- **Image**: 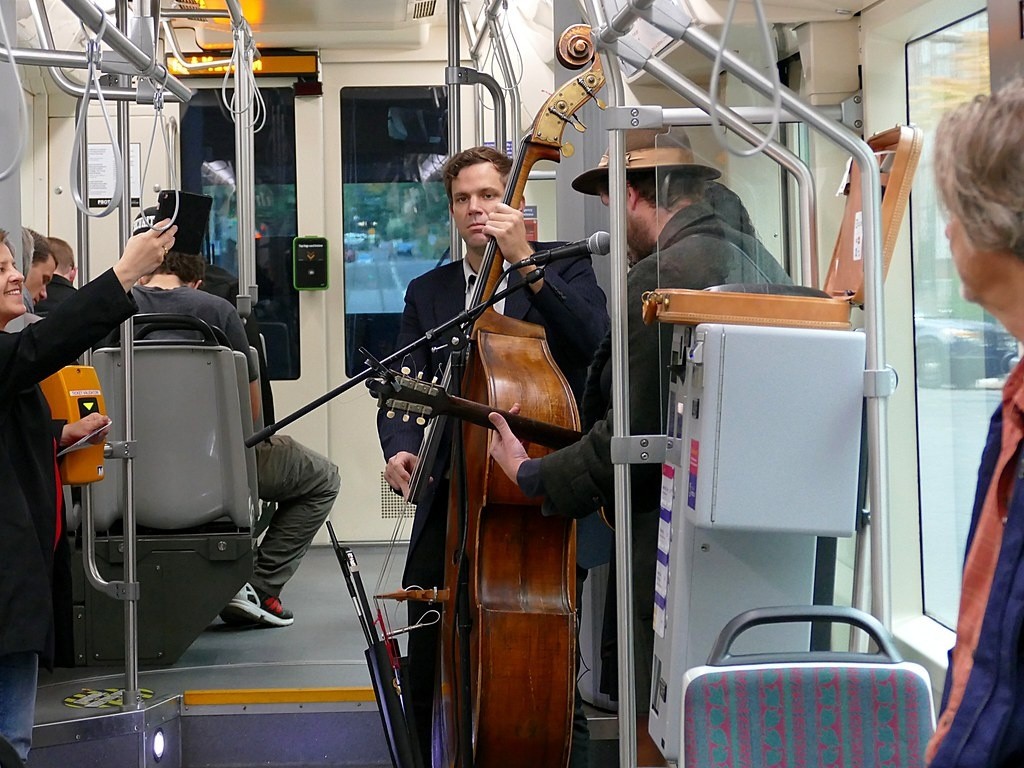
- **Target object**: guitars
[357,344,584,449]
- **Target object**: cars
[912,315,1018,396]
[344,248,358,263]
[342,232,381,250]
[396,244,412,256]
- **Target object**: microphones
[521,231,611,267]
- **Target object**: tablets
[153,190,214,256]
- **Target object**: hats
[570,126,723,196]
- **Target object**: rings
[162,246,168,255]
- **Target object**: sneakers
[220,581,294,627]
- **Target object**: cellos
[431,24,606,767]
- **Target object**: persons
[197,264,276,440]
[0,219,178,768]
[375,146,609,768]
[34,236,78,316]
[487,126,803,768]
[93,251,341,627]
[931,76,1024,768]
[20,227,59,313]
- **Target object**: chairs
[94,312,264,668]
[681,604,940,768]
